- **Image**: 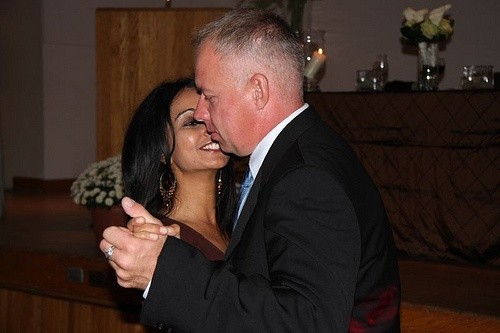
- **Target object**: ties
[232,170,253,230]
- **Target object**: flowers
[68,155,124,210]
[398,3,455,67]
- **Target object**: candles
[303,48,328,81]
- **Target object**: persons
[99,4,401,331]
[120,79,230,333]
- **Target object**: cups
[362,50,387,70]
[354,64,387,93]
[459,65,494,89]
[295,29,326,93]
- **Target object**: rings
[104,246,117,260]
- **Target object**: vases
[416,55,446,90]
[83,206,125,253]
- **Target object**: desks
[0,189,128,309]
[301,90,500,269]
